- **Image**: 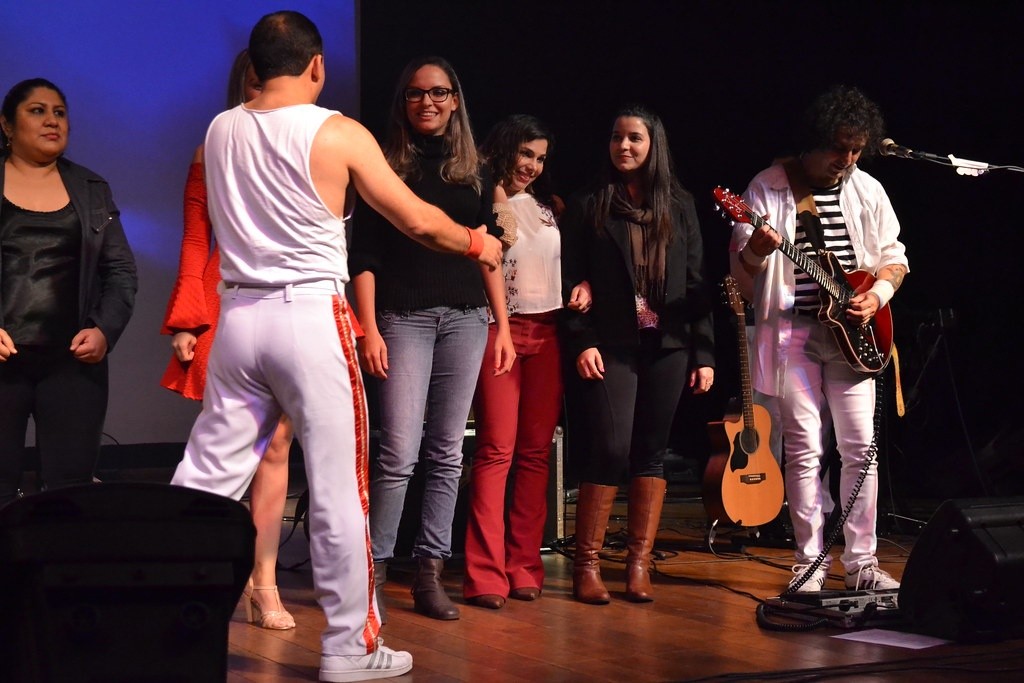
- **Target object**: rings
[706,381,710,384]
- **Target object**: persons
[730,89,907,593]
[465,116,593,608]
[0,79,139,502]
[172,11,503,682]
[164,46,359,631]
[348,55,517,623]
[562,107,716,604]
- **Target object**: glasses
[405,87,453,103]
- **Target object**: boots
[624,477,666,601]
[573,483,618,602]
[411,557,459,620]
[375,563,387,624]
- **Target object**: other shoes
[514,587,540,600]
[472,594,505,609]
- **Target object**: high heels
[243,575,296,629]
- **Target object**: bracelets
[466,226,483,260]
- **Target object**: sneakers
[788,563,829,592]
[845,560,900,591]
[318,637,413,682]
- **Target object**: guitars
[711,181,894,377]
[701,273,785,529]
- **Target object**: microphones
[879,138,924,160]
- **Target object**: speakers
[897,494,1024,639]
[0,481,258,683]
